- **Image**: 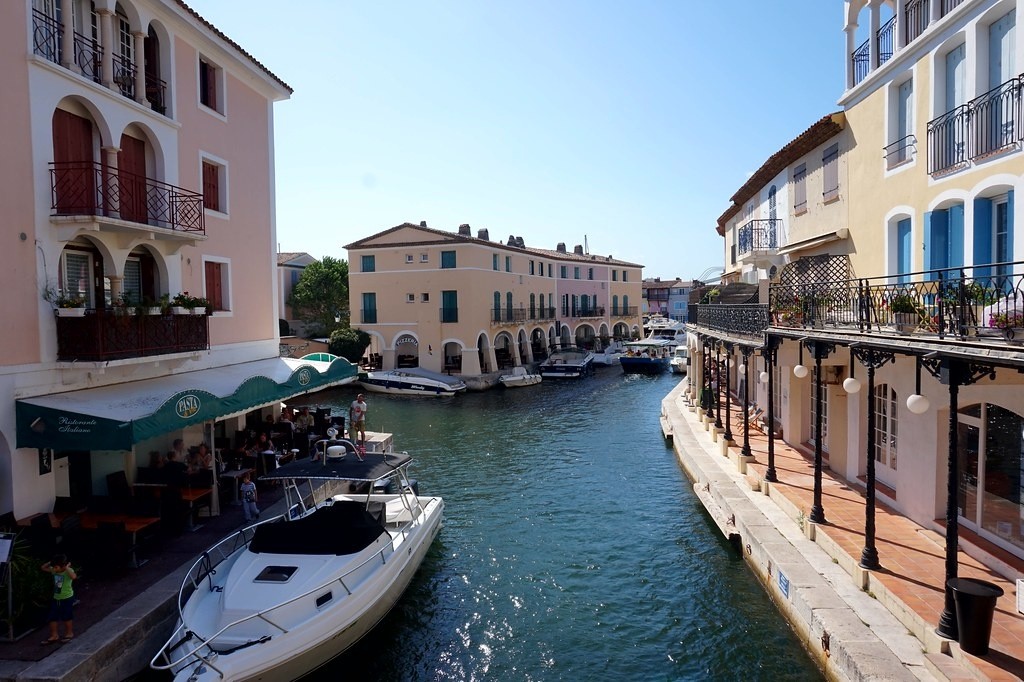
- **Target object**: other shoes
[257,512,260,520]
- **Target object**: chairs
[363,353,419,371]
[738,407,767,436]
[0,405,345,580]
[736,403,759,429]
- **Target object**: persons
[626,348,670,359]
[702,353,728,402]
[41,556,77,645]
[150,393,367,524]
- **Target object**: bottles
[282,441,288,455]
[184,460,188,465]
[188,460,192,472]
[234,457,243,471]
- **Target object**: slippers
[60,633,74,644]
[42,636,60,644]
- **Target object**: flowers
[39,282,87,311]
[114,290,211,316]
[773,278,1024,336]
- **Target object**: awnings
[16,353,359,452]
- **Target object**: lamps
[711,343,715,358]
[907,355,929,413]
[760,357,768,382]
[843,347,861,392]
[739,356,749,374]
[725,351,735,368]
[716,348,724,363]
[705,343,710,354]
[794,342,807,377]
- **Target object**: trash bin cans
[945,577,1004,655]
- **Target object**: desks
[16,513,161,568]
[155,486,211,533]
[191,465,250,506]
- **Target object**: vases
[172,307,190,315]
[57,308,85,317]
[896,313,918,334]
[149,307,162,315]
[113,307,136,315]
[190,307,206,315]
[774,310,802,328]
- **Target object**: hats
[358,394,364,397]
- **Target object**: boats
[590,310,686,373]
[149,424,446,682]
[353,365,468,397]
[536,341,597,379]
[498,366,543,388]
